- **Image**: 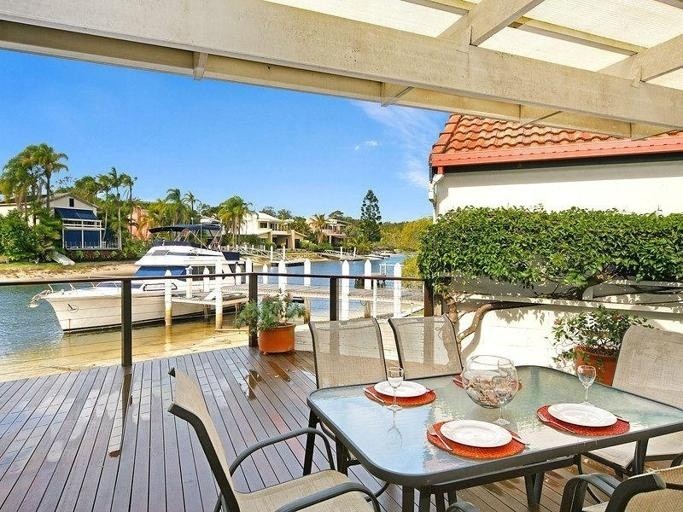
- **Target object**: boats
[41,241,253,334]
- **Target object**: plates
[452,374,523,392]
[548,403,619,428]
[374,381,427,398]
[440,420,512,449]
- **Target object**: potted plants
[543,306,660,387]
[232,288,310,355]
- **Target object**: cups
[387,367,404,411]
[578,365,596,404]
[492,376,512,425]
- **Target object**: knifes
[364,387,385,403]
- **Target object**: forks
[537,413,576,435]
[428,424,453,451]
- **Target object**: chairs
[559,454,683,512]
[302,318,414,512]
[581,326,683,476]
[388,313,467,381]
[168,367,381,512]
